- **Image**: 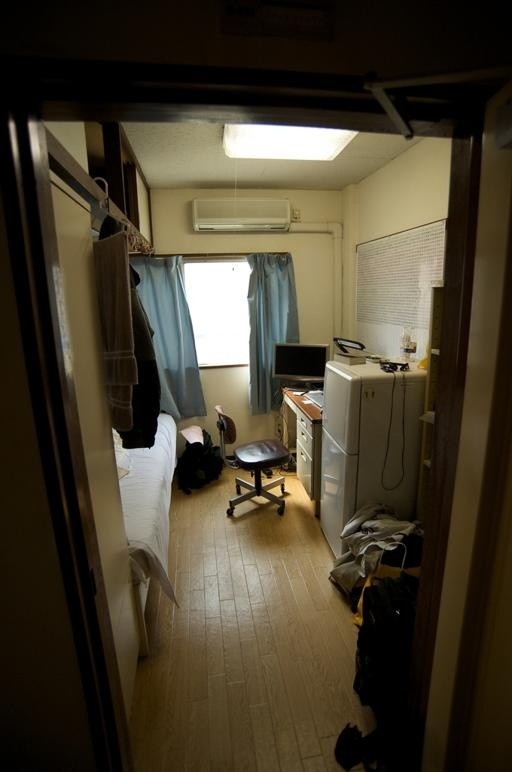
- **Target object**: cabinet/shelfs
[279,386,324,519]
[416,285,445,521]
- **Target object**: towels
[92,230,139,432]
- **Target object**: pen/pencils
[301,391,309,396]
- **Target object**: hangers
[93,200,125,243]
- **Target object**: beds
[111,411,180,657]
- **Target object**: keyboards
[305,393,325,407]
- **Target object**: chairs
[215,404,291,515]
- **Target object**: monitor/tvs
[273,343,330,391]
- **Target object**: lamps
[334,337,365,353]
[221,123,360,162]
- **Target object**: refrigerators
[317,356,426,558]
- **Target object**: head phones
[379,360,410,371]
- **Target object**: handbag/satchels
[175,428,226,496]
[351,541,419,630]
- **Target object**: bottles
[401,324,419,364]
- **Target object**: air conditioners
[191,198,292,233]
